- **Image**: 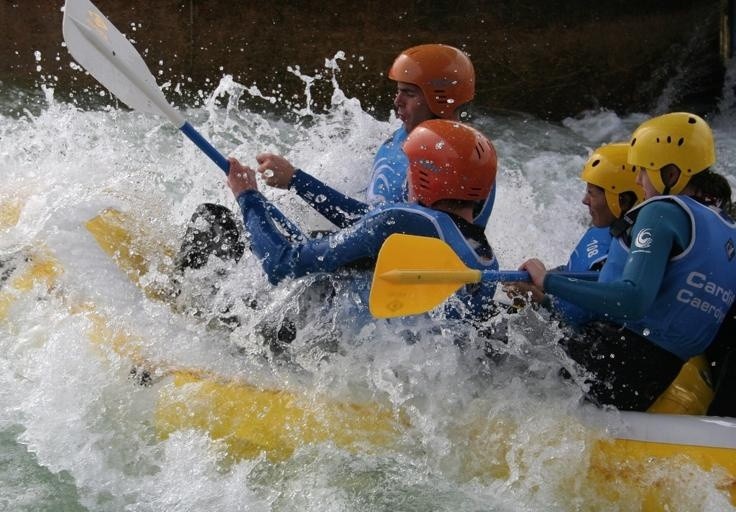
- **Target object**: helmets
[627,110,716,197]
[402,118,498,206]
[390,45,477,118]
[582,143,650,221]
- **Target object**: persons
[502,143,645,323]
[367,44,496,226]
[510,112,736,415]
[170,120,500,362]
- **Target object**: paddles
[62,1,305,242]
[369,233,599,318]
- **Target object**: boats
[0,197,735,512]
